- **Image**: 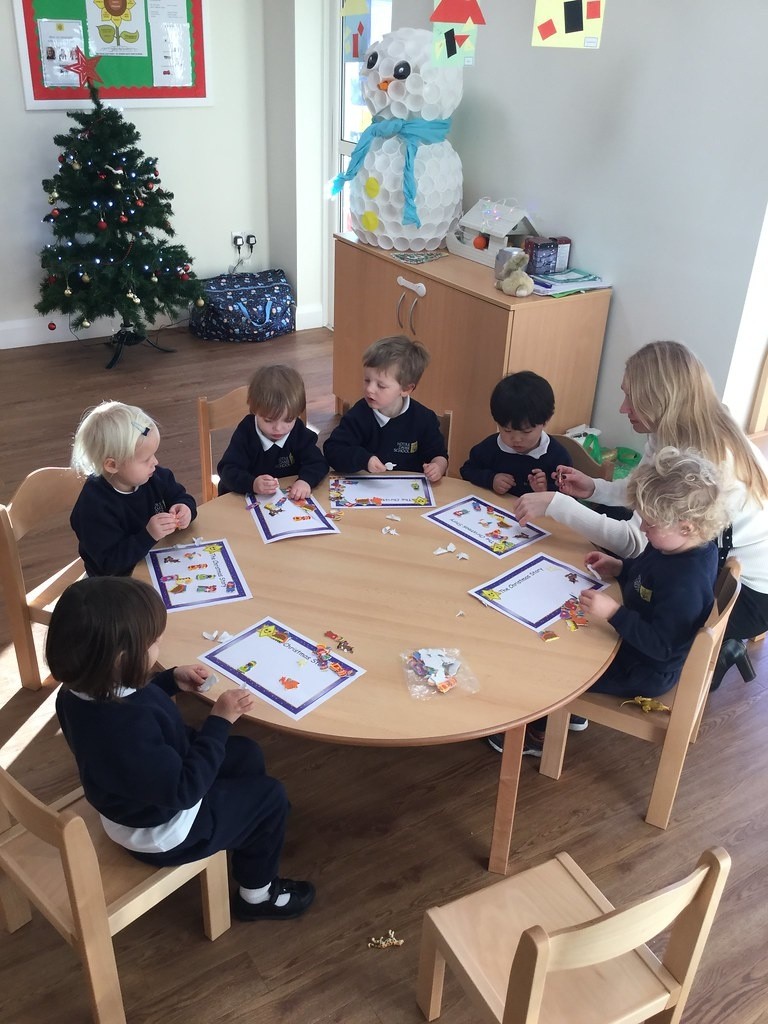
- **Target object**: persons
[323,335,450,482]
[487,447,729,761]
[460,370,573,495]
[59,49,68,60]
[68,401,197,577]
[45,578,315,920]
[513,340,768,641]
[217,363,329,500]
[46,47,55,59]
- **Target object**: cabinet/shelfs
[332,232,613,477]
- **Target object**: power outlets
[243,233,254,245]
[232,229,243,247]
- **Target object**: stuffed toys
[494,250,533,297]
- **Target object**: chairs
[332,399,453,478]
[416,843,732,1024]
[199,374,306,503]
[0,765,232,1024]
[540,557,744,829]
[550,432,614,482]
[0,456,107,691]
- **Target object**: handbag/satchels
[189,269,295,343]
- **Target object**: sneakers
[488,726,544,757]
[532,715,589,732]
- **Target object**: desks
[129,473,625,875]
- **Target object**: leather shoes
[232,876,315,921]
